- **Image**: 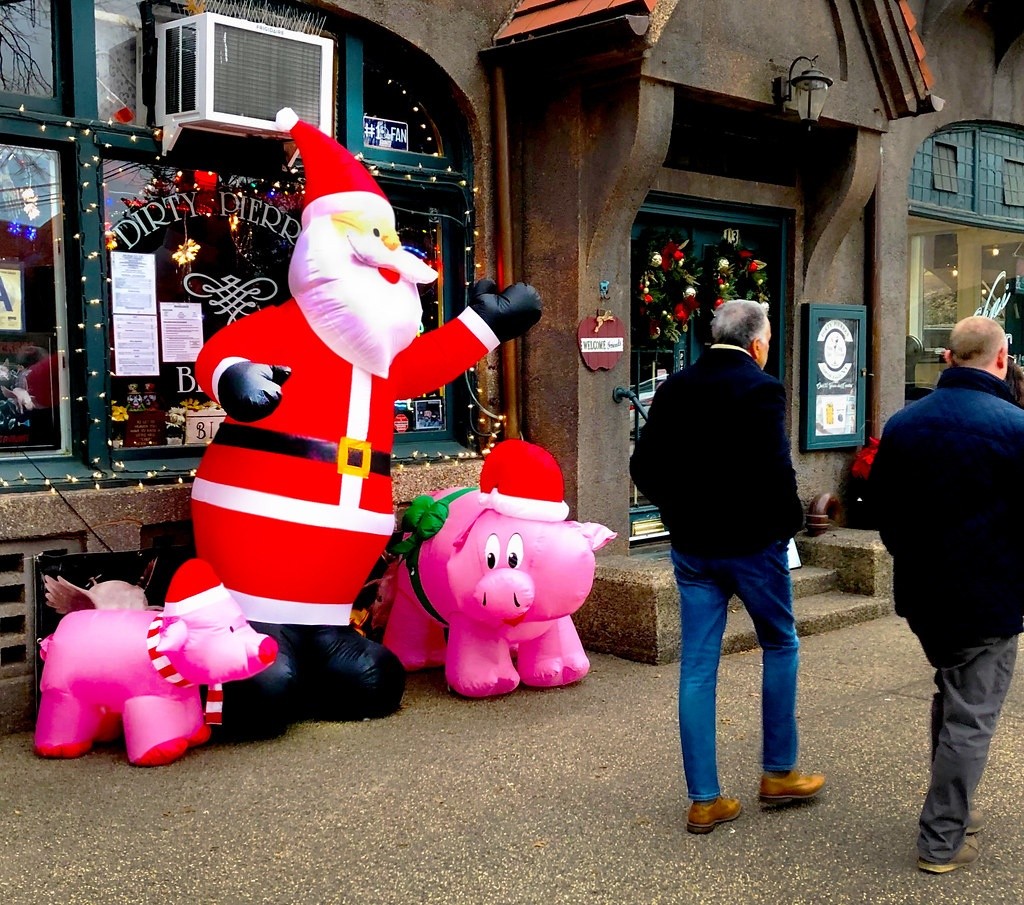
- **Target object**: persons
[630,299,826,835]
[190,107,543,743]
[864,316,1024,875]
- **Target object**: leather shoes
[758,770,825,801]
[687,793,744,834]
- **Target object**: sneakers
[918,836,979,872]
[965,808,985,833]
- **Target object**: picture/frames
[412,396,445,432]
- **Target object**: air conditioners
[155,11,334,140]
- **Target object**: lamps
[773,55,834,132]
